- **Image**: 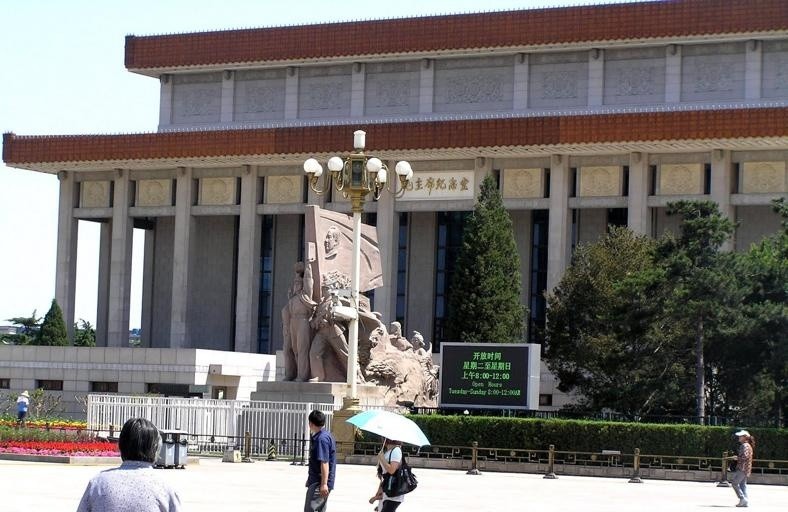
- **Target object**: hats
[734,429,751,437]
[20,390,30,398]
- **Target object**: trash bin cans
[153,429,188,469]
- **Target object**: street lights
[304,130,416,401]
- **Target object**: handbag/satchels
[726,470,737,482]
[381,464,417,497]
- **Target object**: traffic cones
[266,440,277,461]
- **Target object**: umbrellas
[344,408,431,454]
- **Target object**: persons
[731,430,754,507]
[77,417,183,512]
[279,267,441,413]
[368,437,404,512]
[17,390,31,422]
[304,409,336,512]
